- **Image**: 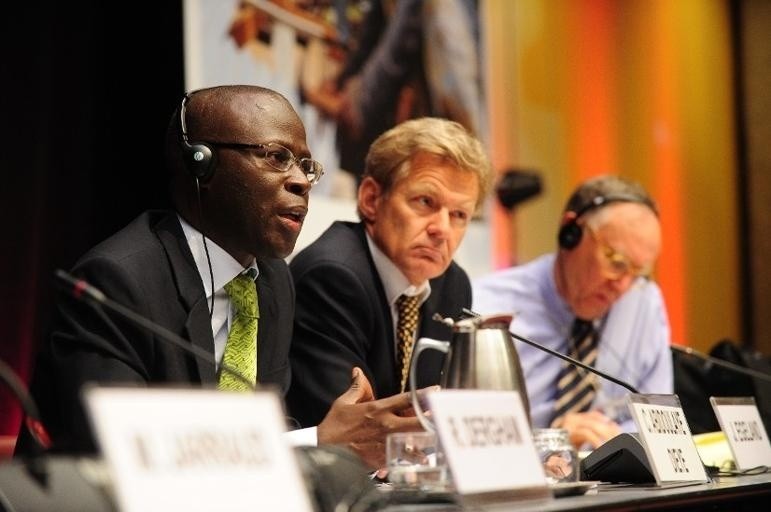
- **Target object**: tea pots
[409,313,532,434]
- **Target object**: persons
[283,116,492,430]
[12,86,444,475]
[472,169,676,452]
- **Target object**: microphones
[51,268,255,390]
[668,343,771,387]
[455,306,642,393]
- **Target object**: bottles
[530,427,580,484]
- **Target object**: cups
[386,431,448,493]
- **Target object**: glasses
[209,139,325,185]
[585,220,651,280]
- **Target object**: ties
[552,319,599,417]
[396,294,422,393]
[217,275,260,391]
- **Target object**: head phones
[168,92,217,182]
[557,192,659,251]
